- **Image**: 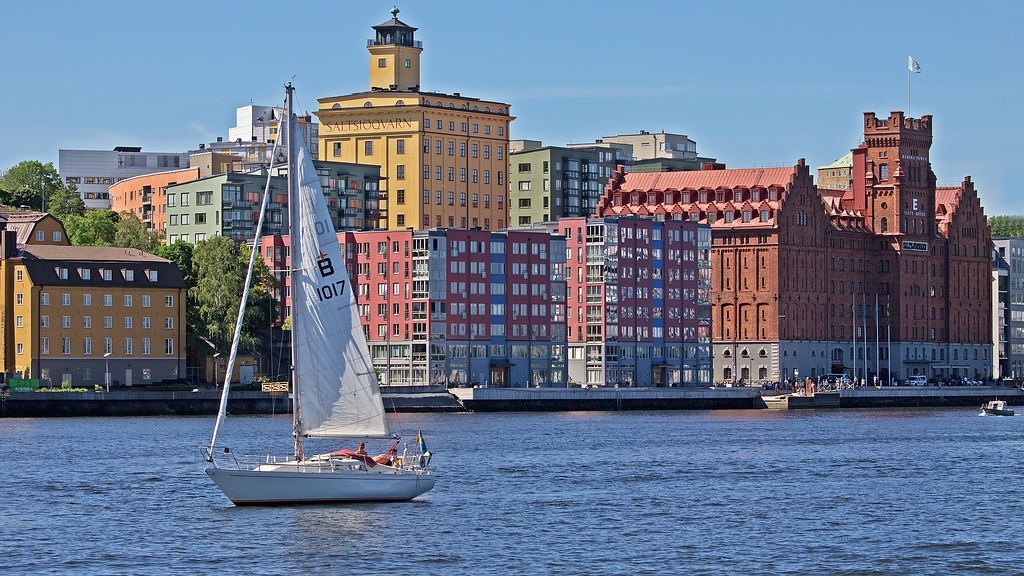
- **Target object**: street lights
[103,352,112,393]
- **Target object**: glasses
[360,447,364,449]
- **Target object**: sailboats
[197,74,437,509]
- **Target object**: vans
[904,375,927,386]
[813,374,852,386]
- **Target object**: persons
[738,373,877,392]
[388,449,403,468]
[356,443,368,456]
[937,376,968,388]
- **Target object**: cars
[942,377,984,386]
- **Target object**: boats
[980,397,1014,416]
[761,379,840,410]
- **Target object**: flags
[907,55,922,73]
